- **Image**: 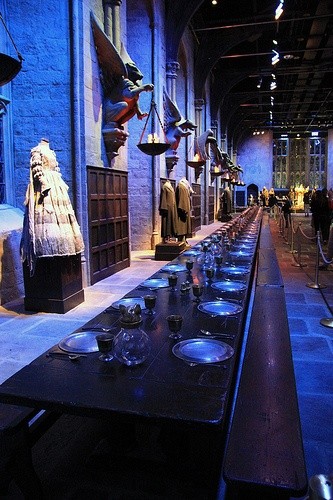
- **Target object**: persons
[159,176,193,248]
[303,188,333,270]
[20,138,86,276]
[248,185,295,227]
[220,187,232,214]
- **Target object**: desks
[0,213,263,500]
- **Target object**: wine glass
[168,275,179,291]
[185,261,194,274]
[201,207,259,253]
[213,256,223,267]
[205,269,215,283]
[166,314,183,339]
[143,295,157,314]
[192,283,204,303]
[96,332,115,362]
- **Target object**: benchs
[257,216,283,285]
[224,286,306,495]
[0,402,60,500]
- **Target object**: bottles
[112,303,152,367]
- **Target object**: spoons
[200,329,235,337]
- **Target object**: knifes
[49,351,88,358]
[196,335,233,339]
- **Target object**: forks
[185,362,227,368]
[81,327,111,331]
[46,354,80,360]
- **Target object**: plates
[210,281,248,292]
[161,264,187,271]
[111,298,146,310]
[59,332,119,354]
[235,244,254,250]
[139,279,169,288]
[172,338,235,363]
[219,267,250,275]
[230,252,253,257]
[183,251,203,257]
[197,301,243,315]
